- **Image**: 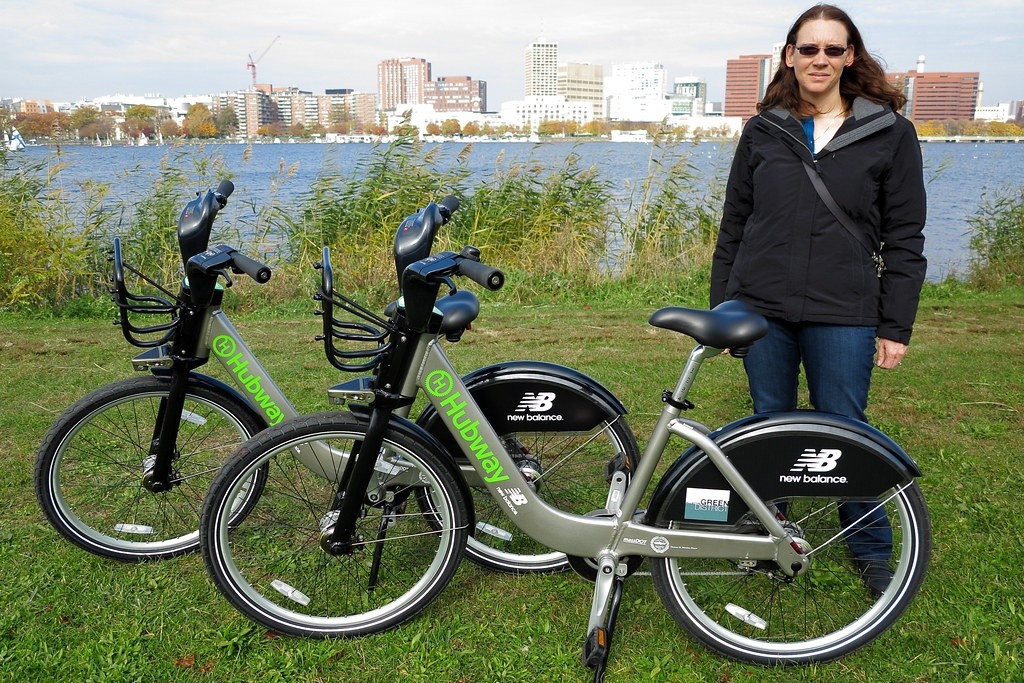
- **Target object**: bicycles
[34,177,641,596]
[201,195,934,682]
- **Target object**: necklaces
[814,111,845,142]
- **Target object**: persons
[709,4,928,600]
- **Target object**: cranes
[244,33,279,91]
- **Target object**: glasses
[792,44,850,56]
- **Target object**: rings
[899,362,902,366]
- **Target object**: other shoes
[858,559,894,599]
[752,556,779,571]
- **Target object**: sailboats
[0,119,165,152]
